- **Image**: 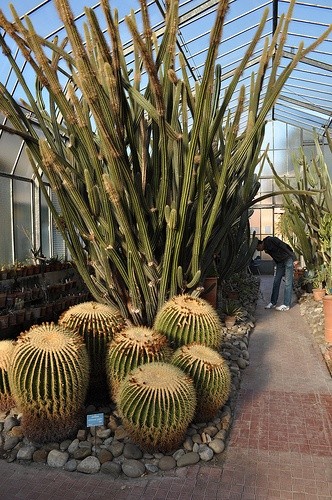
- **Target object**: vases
[202,275,218,309]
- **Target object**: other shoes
[275,305,290,311]
[264,303,276,309]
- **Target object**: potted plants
[266,126,332,345]
[220,299,243,328]
[1,259,93,331]
[225,281,241,300]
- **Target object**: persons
[255,236,297,311]
